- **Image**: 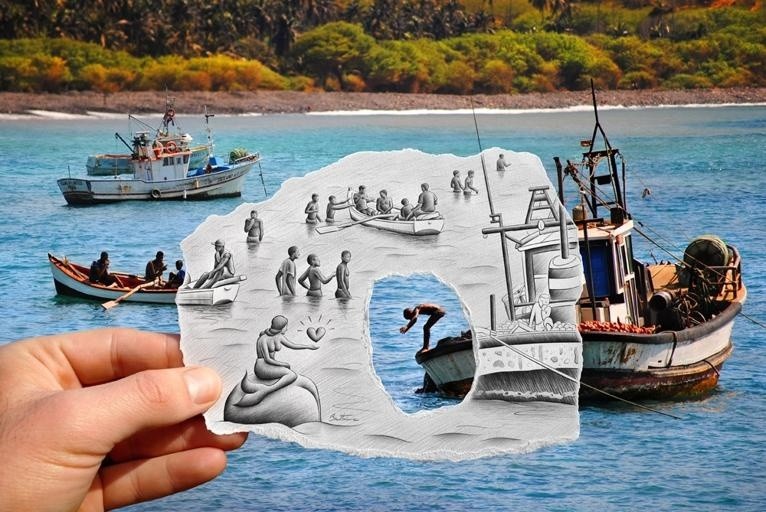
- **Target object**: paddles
[101,279,159,309]
[315,213,399,234]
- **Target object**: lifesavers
[154,140,179,155]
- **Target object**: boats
[416,78,749,402]
[82,142,218,174]
[346,187,445,237]
[49,251,239,305]
[57,112,262,206]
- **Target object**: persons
[145,251,168,288]
[0,327,249,512]
[95,252,124,288]
[165,260,186,289]
